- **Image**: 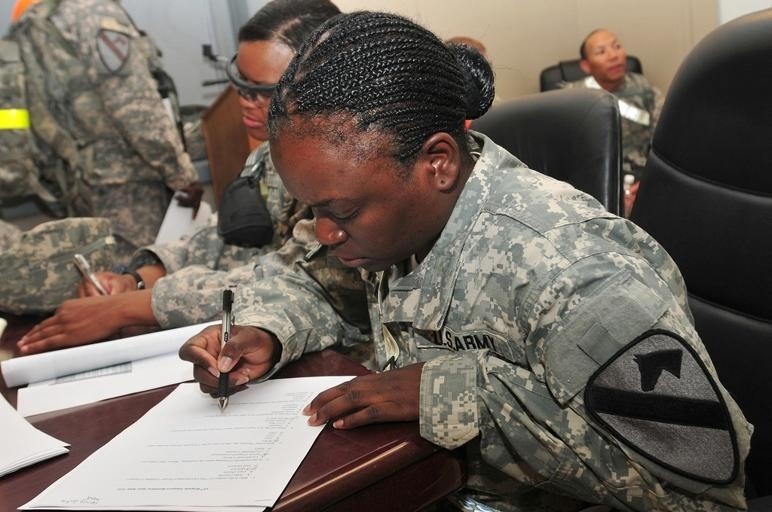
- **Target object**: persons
[176,8,759,512]
[0,1,341,354]
[555,27,667,222]
[443,37,503,130]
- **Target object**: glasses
[226,53,283,105]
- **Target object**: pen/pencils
[217,289,235,413]
[73,253,109,295]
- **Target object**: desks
[0,315,459,512]
